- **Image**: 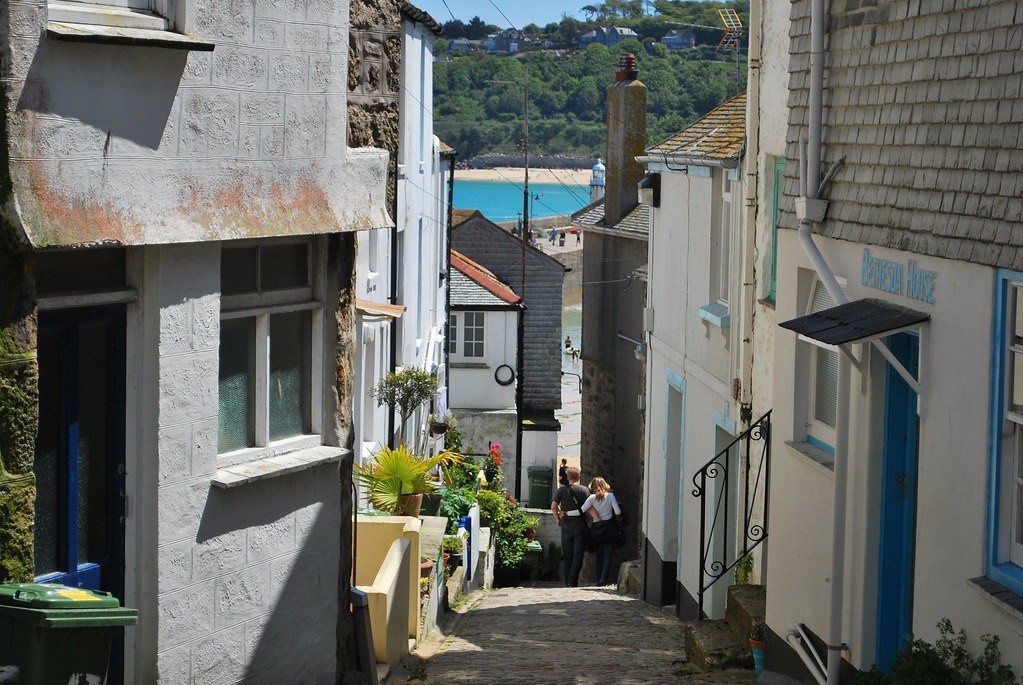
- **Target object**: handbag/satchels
[617,528,626,548]
[586,530,598,553]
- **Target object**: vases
[483,469,496,482]
[421,597,425,609]
[430,423,449,433]
[499,509,513,528]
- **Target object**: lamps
[636,173,660,208]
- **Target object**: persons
[528,229,534,242]
[551,467,621,587]
[560,229,565,241]
[549,227,557,245]
[559,459,569,488]
[576,231,580,246]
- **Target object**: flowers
[428,410,460,427]
[477,443,504,475]
[497,486,520,511]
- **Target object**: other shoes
[599,582,607,586]
[566,578,577,587]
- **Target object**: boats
[566,346,576,355]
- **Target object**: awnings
[451,244,520,305]
[776,298,926,347]
[359,295,405,320]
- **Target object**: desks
[523,538,542,581]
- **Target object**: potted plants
[367,366,439,516]
[494,513,525,586]
[420,476,444,516]
[523,514,541,542]
[420,577,428,598]
[477,490,504,526]
[445,525,470,577]
[442,537,450,567]
[749,615,766,676]
[421,554,434,577]
[443,564,452,586]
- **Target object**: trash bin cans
[0,581,138,685]
[525,465,553,509]
